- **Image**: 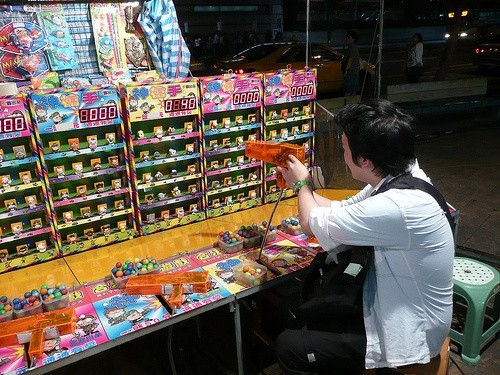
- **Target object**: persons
[184,31,300,60]
[276,98,455,375]
[341,31,360,97]
[404,33,424,83]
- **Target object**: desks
[0,216,326,375]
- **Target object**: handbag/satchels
[407,66,424,78]
[341,63,351,74]
[301,251,368,331]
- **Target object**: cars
[190,40,392,92]
[443,25,478,40]
[471,32,500,68]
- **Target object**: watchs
[293,180,314,194]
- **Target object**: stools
[449,257,500,366]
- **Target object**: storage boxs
[262,227,277,243]
[216,235,243,254]
[15,303,42,318]
[0,311,15,323]
[110,270,139,288]
[281,220,305,236]
[41,288,69,311]
[244,235,261,247]
[140,261,160,276]
[232,260,267,286]
